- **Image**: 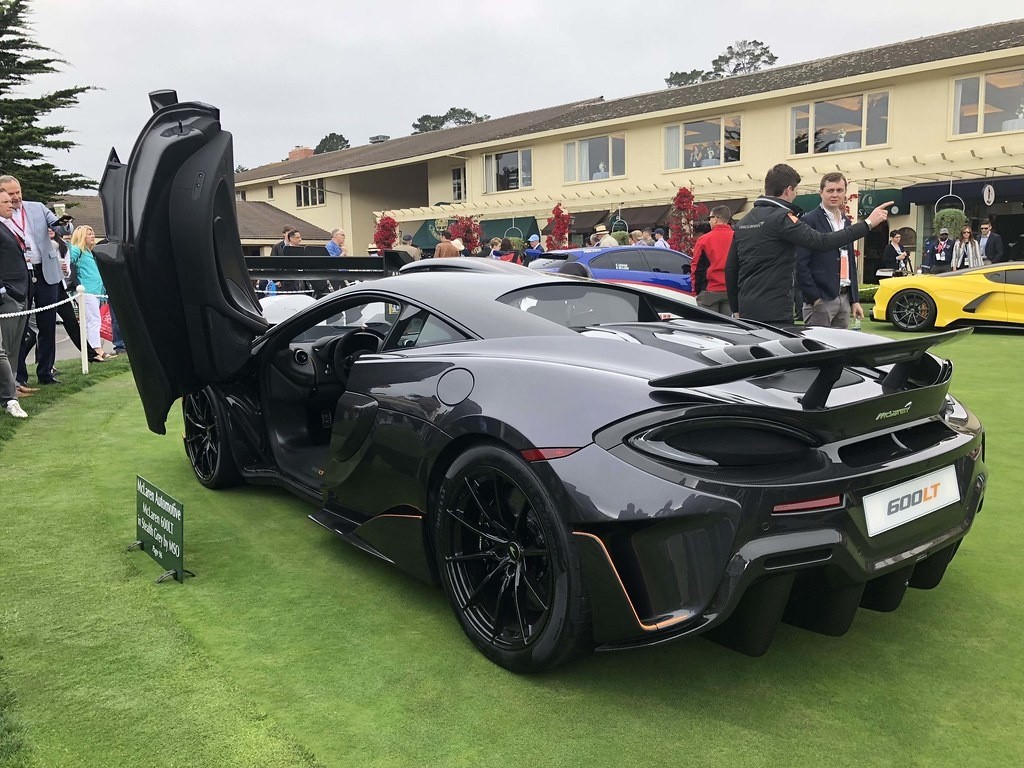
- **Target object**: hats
[632,230,643,240]
[596,225,610,234]
[528,234,539,242]
[940,228,949,235]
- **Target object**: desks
[593,172,610,180]
[1002,119,1024,131]
[829,142,860,151]
[702,159,720,166]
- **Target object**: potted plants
[933,208,968,241]
[507,237,528,250]
[610,230,629,245]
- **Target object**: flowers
[1015,104,1024,115]
[708,148,715,155]
[599,162,606,170]
[837,129,846,137]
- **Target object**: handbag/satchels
[66,250,82,292]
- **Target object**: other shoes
[17,386,40,392]
[5,400,28,418]
[104,353,118,359]
[16,390,31,398]
[51,378,63,385]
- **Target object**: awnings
[411,216,541,249]
[901,175,1024,205]
[791,187,910,219]
[541,198,746,235]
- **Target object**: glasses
[707,216,719,221]
[294,236,301,239]
[981,228,988,230]
[963,231,971,234]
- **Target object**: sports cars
[90,85,990,674]
[870,260,1024,334]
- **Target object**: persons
[794,172,864,329]
[325,228,348,257]
[392,234,421,260]
[527,234,544,252]
[0,175,127,419]
[271,224,301,256]
[976,221,1004,265]
[926,228,955,274]
[433,232,459,258]
[882,230,907,271]
[691,205,733,317]
[724,163,894,324]
[590,225,618,247]
[477,237,522,264]
[642,228,655,246]
[950,225,984,271]
[627,230,647,246]
[654,228,670,248]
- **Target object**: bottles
[851,317,861,332]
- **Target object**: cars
[493,245,697,320]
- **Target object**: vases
[600,169,604,172]
[709,155,712,159]
[1018,114,1023,119]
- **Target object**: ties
[5,221,26,250]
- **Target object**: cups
[899,253,904,256]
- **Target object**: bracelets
[865,219,873,231]
